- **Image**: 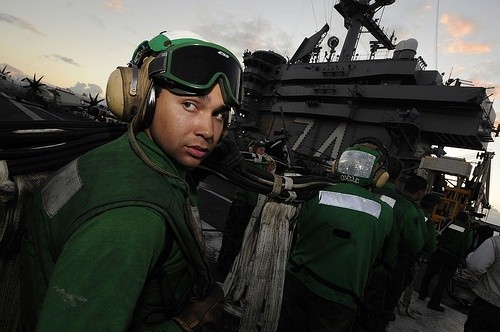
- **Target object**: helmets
[336,137,386,185]
[129,34,237,126]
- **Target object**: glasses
[148,42,244,108]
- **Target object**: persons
[352,157,425,332]
[463,231,500,332]
[236,139,276,205]
[282,138,401,332]
[376,176,440,332]
[16,30,244,332]
[418,211,471,312]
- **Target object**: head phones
[333,138,390,188]
[248,139,263,154]
[106,30,210,122]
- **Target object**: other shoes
[427,300,445,313]
[418,294,425,301]
